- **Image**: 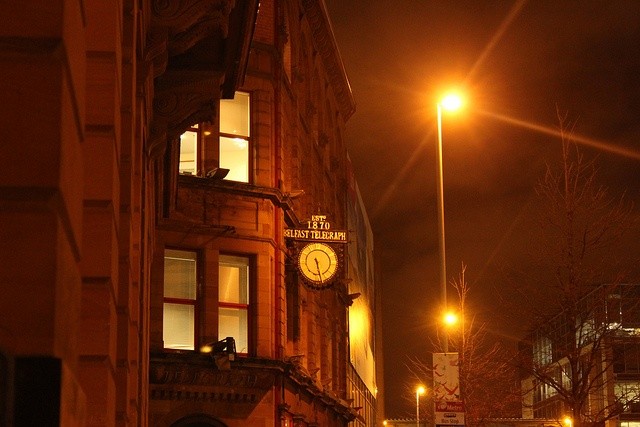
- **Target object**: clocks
[299,243,337,289]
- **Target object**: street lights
[417,385,427,427]
[435,88,467,354]
